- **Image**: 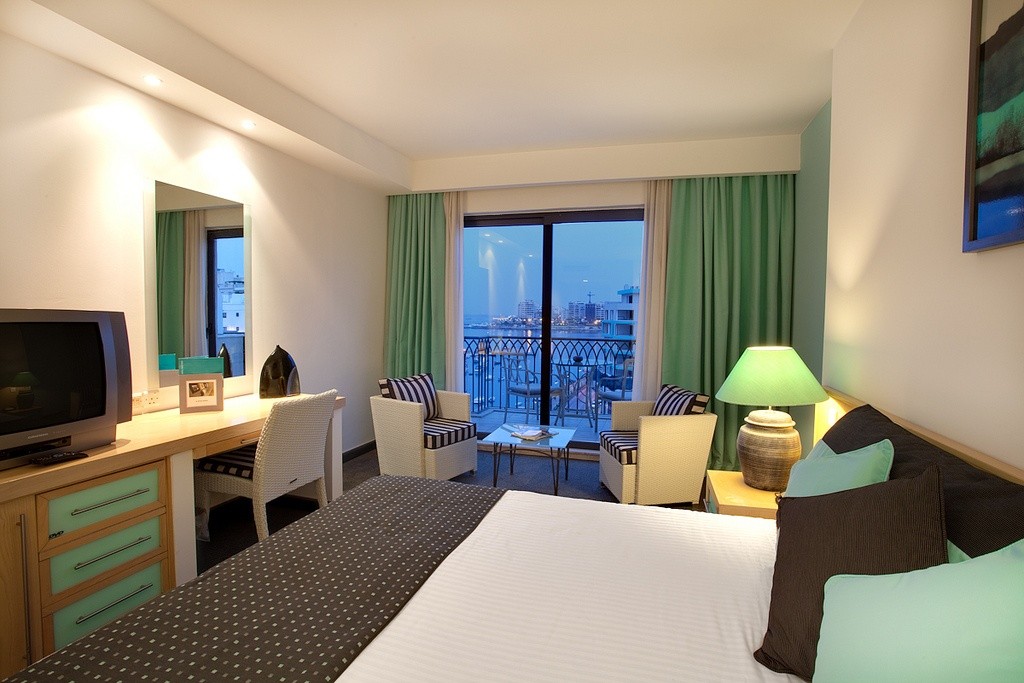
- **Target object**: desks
[0,392,347,681]
[547,358,614,429]
[483,423,578,496]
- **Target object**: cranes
[587,291,599,303]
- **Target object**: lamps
[713,346,830,493]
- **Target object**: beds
[0,385,1024,683]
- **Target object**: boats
[515,402,522,406]
[488,396,496,402]
[474,397,484,404]
[464,349,500,376]
[498,377,504,381]
[486,375,491,380]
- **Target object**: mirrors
[142,178,254,416]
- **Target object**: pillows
[780,437,893,500]
[812,538,1024,683]
[753,463,949,683]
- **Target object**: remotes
[31,452,87,465]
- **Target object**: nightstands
[703,469,787,520]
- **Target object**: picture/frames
[178,372,223,414]
[962,0,1024,255]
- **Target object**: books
[511,429,559,441]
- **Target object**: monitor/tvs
[0,309,133,471]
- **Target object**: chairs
[502,347,565,427]
[599,383,718,507]
[191,389,339,545]
[368,372,477,482]
[594,358,636,433]
